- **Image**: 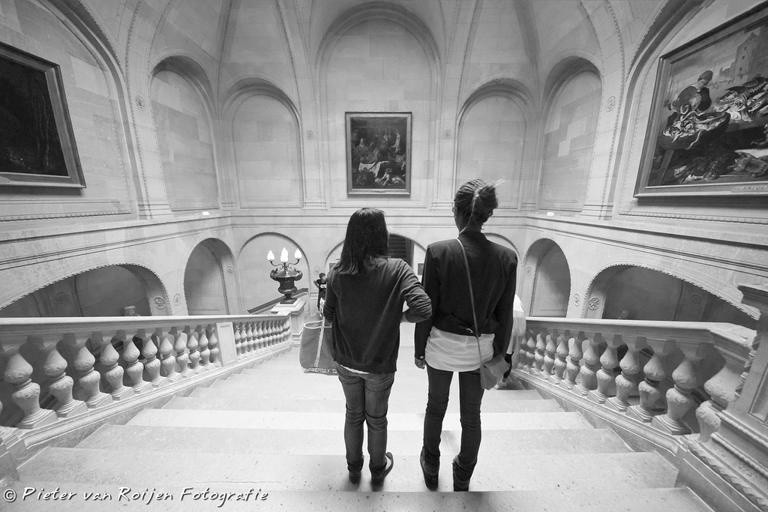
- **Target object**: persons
[313,271,329,311]
[662,68,713,119]
[323,207,433,483]
[413,177,520,494]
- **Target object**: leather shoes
[349,471,361,483]
[372,452,393,480]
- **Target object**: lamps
[267,247,303,304]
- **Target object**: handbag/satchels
[480,354,510,389]
[300,321,338,375]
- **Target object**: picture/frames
[344,111,413,195]
[632,1,768,198]
[0,41,87,190]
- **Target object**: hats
[700,70,712,81]
